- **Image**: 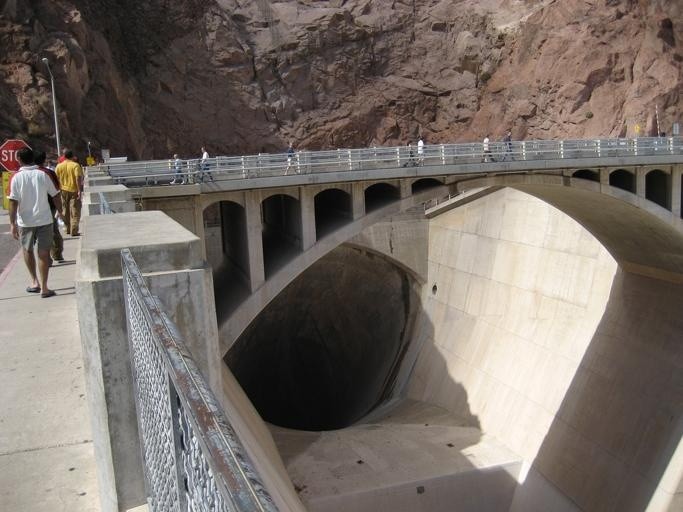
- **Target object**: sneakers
[65,232,80,236]
[41,290,56,298]
[169,182,187,185]
[26,286,39,292]
[48,258,65,267]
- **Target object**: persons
[501,131,515,161]
[6,148,84,298]
[284,143,298,175]
[403,141,418,167]
[481,134,495,162]
[169,154,186,185]
[655,132,670,151]
[417,136,424,166]
[199,146,214,182]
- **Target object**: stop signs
[0,138,33,173]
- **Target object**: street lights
[41,57,62,157]
